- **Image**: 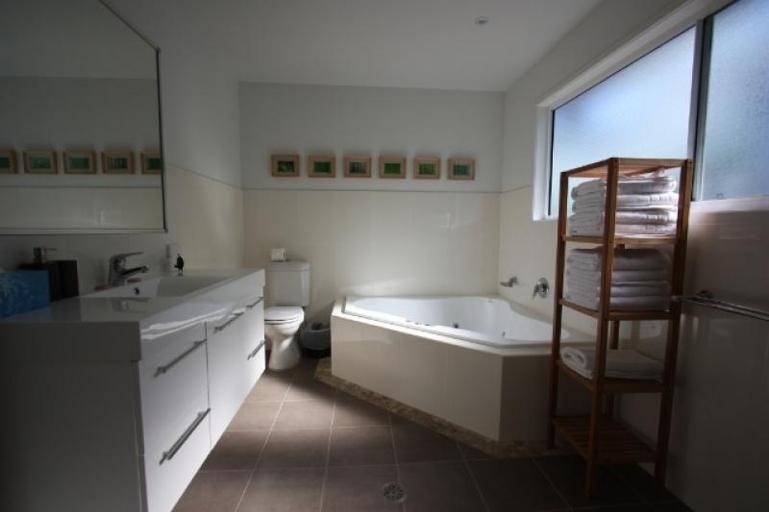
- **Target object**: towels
[562,173,678,379]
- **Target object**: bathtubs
[330,294,599,442]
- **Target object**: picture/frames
[413,156,441,180]
[62,149,98,175]
[378,155,407,179]
[102,150,134,175]
[344,155,372,178]
[307,154,337,177]
[24,148,58,176]
[139,151,162,175]
[1,149,19,175]
[270,154,300,178]
[447,158,477,180]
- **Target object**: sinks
[80,274,232,299]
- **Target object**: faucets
[110,251,149,285]
[531,283,547,300]
[33,247,59,263]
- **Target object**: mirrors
[0,1,170,236]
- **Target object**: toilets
[263,261,311,371]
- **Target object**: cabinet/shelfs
[207,286,267,451]
[548,158,696,512]
[1,323,211,512]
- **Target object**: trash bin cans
[299,321,331,363]
